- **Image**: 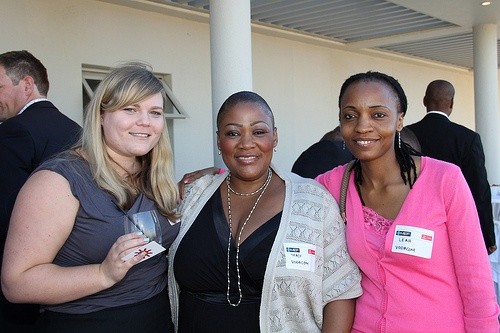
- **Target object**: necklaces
[226,167,273,307]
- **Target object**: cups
[124,210,163,263]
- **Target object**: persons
[313,71,499,333]
[167,90,363,333]
[291,124,354,178]
[401,79,497,256]
[1,64,175,333]
[0,50,84,257]
